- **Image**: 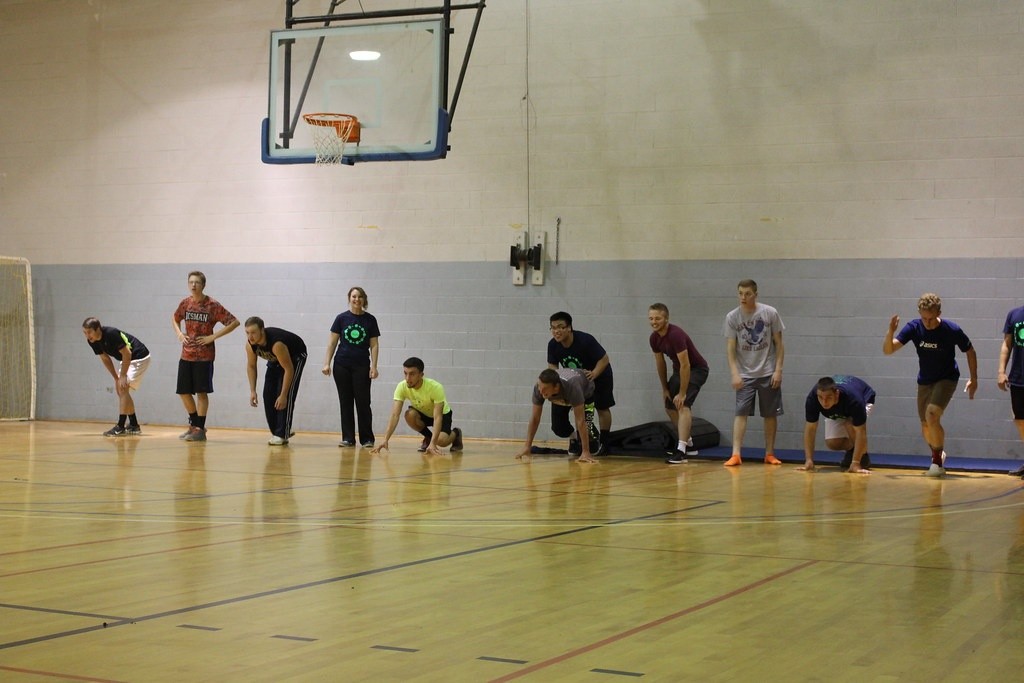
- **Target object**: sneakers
[666,445,698,456]
[925,463,946,477]
[450,427,463,451]
[268,435,289,445]
[339,440,355,447]
[860,452,870,469]
[364,441,373,447]
[185,427,206,441]
[841,446,855,472]
[664,449,688,464]
[932,450,946,467]
[103,423,128,437]
[124,424,141,434]
[568,430,582,455]
[287,431,295,439]
[590,431,602,455]
[179,425,196,439]
[421,433,432,449]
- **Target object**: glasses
[549,325,569,332]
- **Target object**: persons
[997,305,1024,480]
[648,303,710,463]
[172,271,240,441]
[245,316,308,445]
[884,293,977,478]
[514,368,602,463]
[548,311,616,456]
[370,357,464,455]
[82,317,151,437]
[722,279,786,465]
[793,376,877,475]
[322,287,382,447]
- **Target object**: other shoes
[596,444,608,458]
[1009,465,1024,480]
